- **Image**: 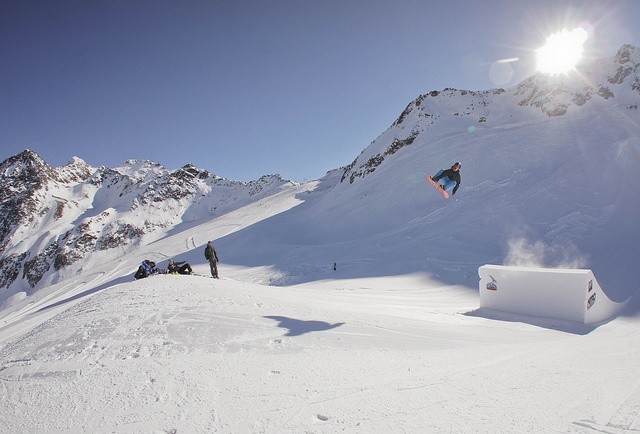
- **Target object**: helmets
[454,163,462,168]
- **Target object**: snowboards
[424,175,450,199]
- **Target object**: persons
[133,259,156,281]
[204,240,220,279]
[431,162,462,197]
[167,258,196,276]
